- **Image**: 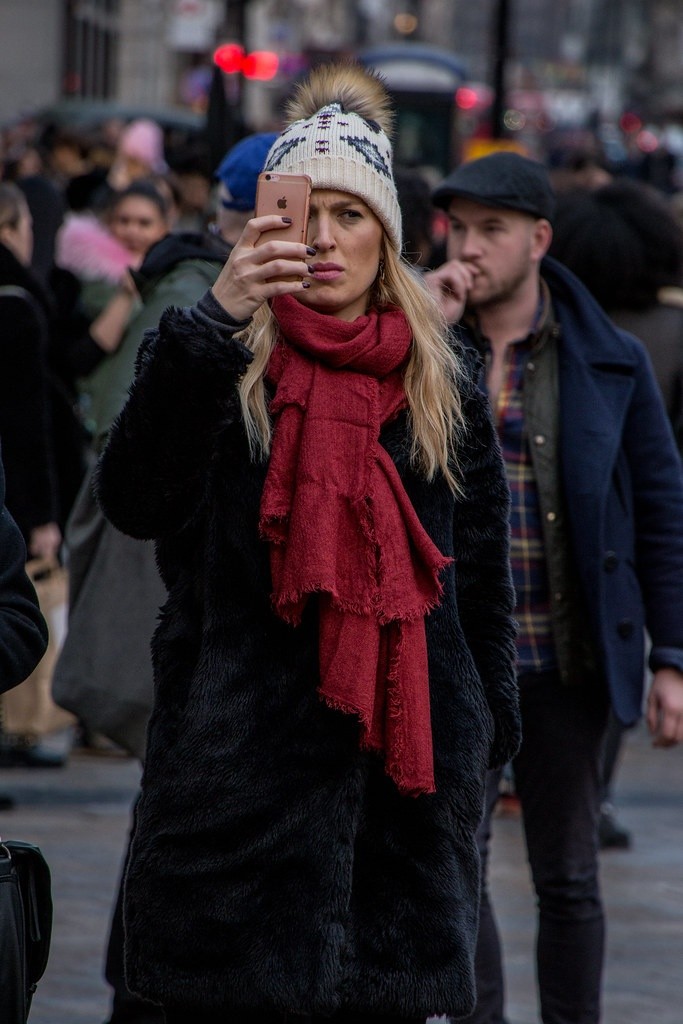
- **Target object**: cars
[42,98,209,169]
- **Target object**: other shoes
[595,800,628,849]
[495,778,520,813]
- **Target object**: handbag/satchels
[5,559,77,735]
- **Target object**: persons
[0,103,683,1024]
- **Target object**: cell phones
[255,171,311,283]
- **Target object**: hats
[430,151,556,222]
[118,118,171,178]
[261,58,403,259]
[214,133,280,214]
